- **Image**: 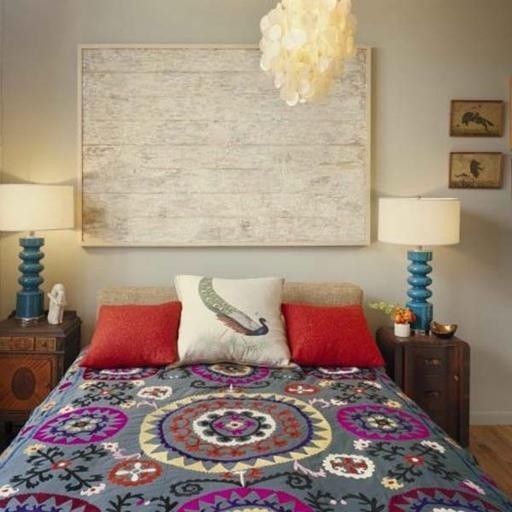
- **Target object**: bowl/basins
[431,325,457,339]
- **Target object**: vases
[393,323,411,338]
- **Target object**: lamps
[254,0,356,107]
[0,181,77,326]
[377,195,463,333]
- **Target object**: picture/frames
[73,43,373,250]
[448,150,505,190]
[448,98,505,138]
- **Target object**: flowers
[367,300,417,324]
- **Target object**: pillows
[78,298,179,372]
[280,298,387,366]
[169,272,294,371]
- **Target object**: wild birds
[198,276,269,346]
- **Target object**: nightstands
[378,327,471,455]
[0,307,79,426]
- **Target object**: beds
[0,283,512,511]
